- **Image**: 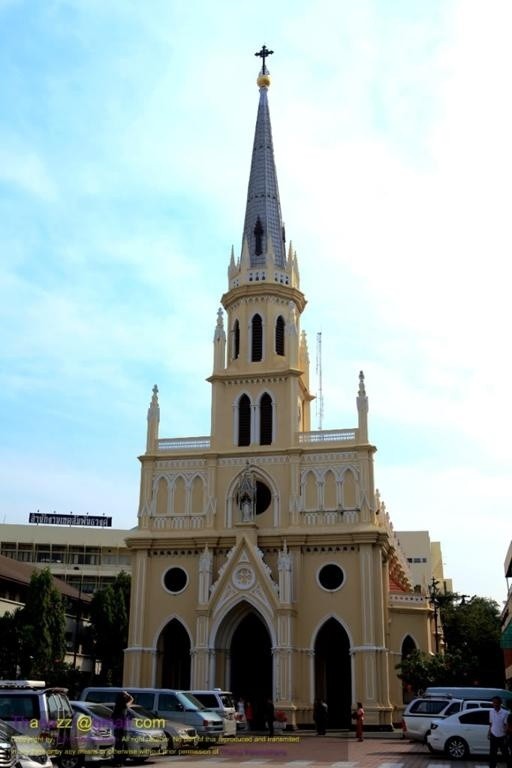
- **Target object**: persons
[504,698,511,767]
[486,694,511,767]
[230,694,275,739]
[111,689,136,767]
[353,700,365,743]
[313,698,330,735]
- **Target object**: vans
[0,681,249,768]
[401,687,512,741]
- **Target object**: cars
[427,708,512,757]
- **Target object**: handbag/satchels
[352,711,357,720]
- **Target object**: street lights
[73,567,83,670]
